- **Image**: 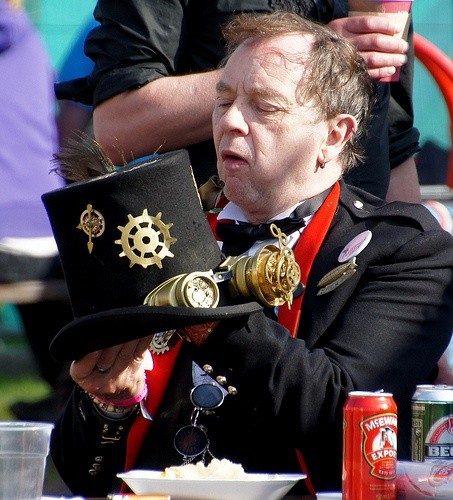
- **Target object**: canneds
[394,460,453,500]
[412,384,453,465]
[341,388,397,500]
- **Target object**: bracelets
[85,383,152,420]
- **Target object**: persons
[0,0,66,239]
[53,18,100,153]
[84,0,423,206]
[48,9,453,500]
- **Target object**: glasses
[171,382,224,458]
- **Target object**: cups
[350,1,412,82]
[315,493,342,500]
[0,422,55,500]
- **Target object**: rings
[96,366,110,373]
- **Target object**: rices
[161,458,254,480]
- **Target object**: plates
[115,472,308,500]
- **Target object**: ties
[215,185,334,256]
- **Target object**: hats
[40,150,302,362]
